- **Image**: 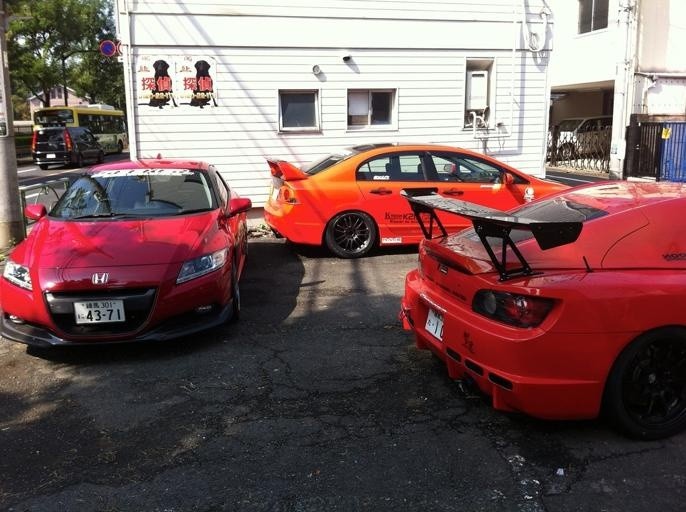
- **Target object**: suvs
[31,126,107,170]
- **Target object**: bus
[32,103,129,155]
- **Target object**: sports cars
[0,159,251,350]
[396,179,686,441]
[263,142,574,259]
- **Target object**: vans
[547,114,611,160]
